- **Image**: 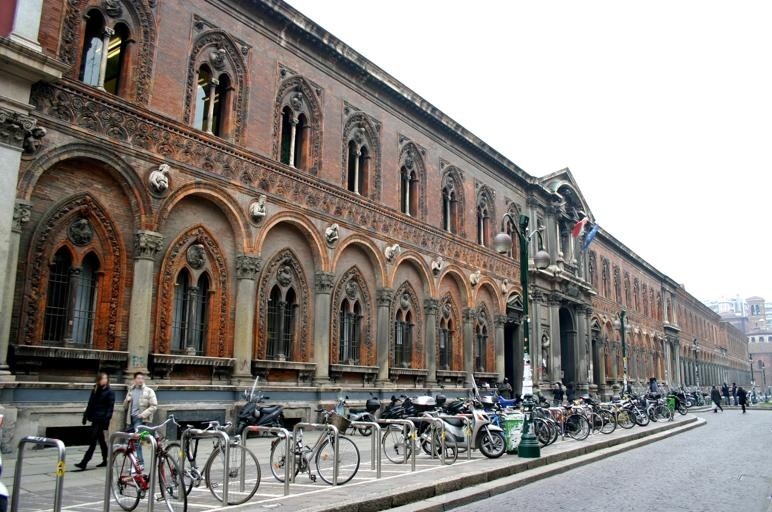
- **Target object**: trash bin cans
[501,410,524,455]
[667,395,675,411]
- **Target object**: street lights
[748,353,768,394]
[614,308,631,391]
[495,214,551,434]
[691,338,700,391]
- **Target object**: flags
[572,217,589,239]
[582,224,598,251]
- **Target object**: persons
[731,382,738,405]
[72,371,116,470]
[736,387,748,413]
[710,385,723,412]
[497,374,512,400]
[532,384,547,408]
[122,371,159,474]
[566,379,578,405]
[552,382,564,408]
[721,382,730,407]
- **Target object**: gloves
[82,417,86,424]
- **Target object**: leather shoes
[74,463,86,469]
[96,462,108,467]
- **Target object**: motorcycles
[237,393,284,435]
[336,391,506,463]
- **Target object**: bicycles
[499,390,695,449]
[111,415,260,512]
[269,408,360,485]
